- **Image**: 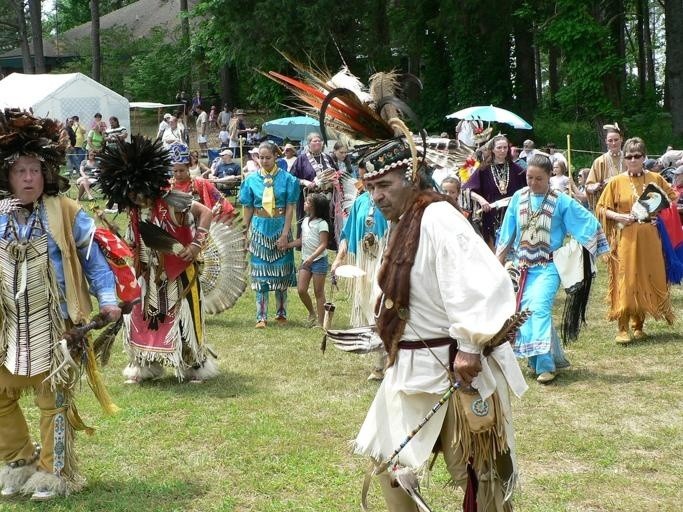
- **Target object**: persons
[349,138,530,512]
[1,86,390,385]
[1,108,122,506]
[430,117,683,387]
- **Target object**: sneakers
[631,329,647,340]
[366,370,385,382]
[615,330,634,345]
[256,321,266,329]
[537,370,557,382]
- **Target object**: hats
[163,113,173,118]
[358,142,427,178]
[219,149,233,156]
[249,147,259,154]
[234,108,247,116]
[167,143,191,164]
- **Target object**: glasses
[624,154,642,160]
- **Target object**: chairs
[74,148,240,205]
